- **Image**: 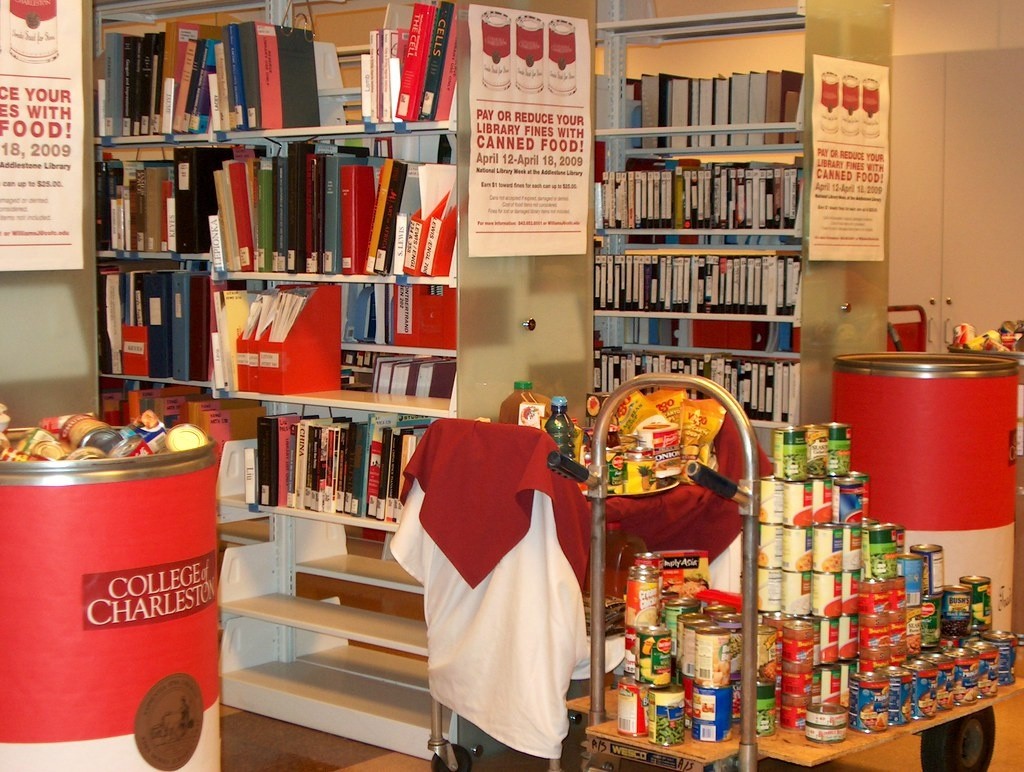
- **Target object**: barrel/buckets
[832,352,1020,632]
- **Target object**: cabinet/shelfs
[595,0,896,450]
[88,0,593,763]
[891,42,1023,352]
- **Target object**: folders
[98,269,211,383]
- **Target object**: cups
[820,72,880,138]
[482,10,577,96]
[9,0,59,64]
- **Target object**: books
[596,70,807,148]
[89,142,455,278]
[95,0,460,136]
[593,254,802,316]
[100,377,443,526]
[593,141,808,245]
[593,315,793,353]
[592,348,799,424]
[96,270,459,400]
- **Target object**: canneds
[0,411,208,462]
[617,423,1020,748]
[604,447,658,495]
[953,321,1024,354]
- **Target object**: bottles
[499,381,582,463]
[607,424,623,496]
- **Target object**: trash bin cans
[0,426,223,772]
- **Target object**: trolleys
[568,373,1024,772]
[426,422,630,772]
[888,305,927,354]
